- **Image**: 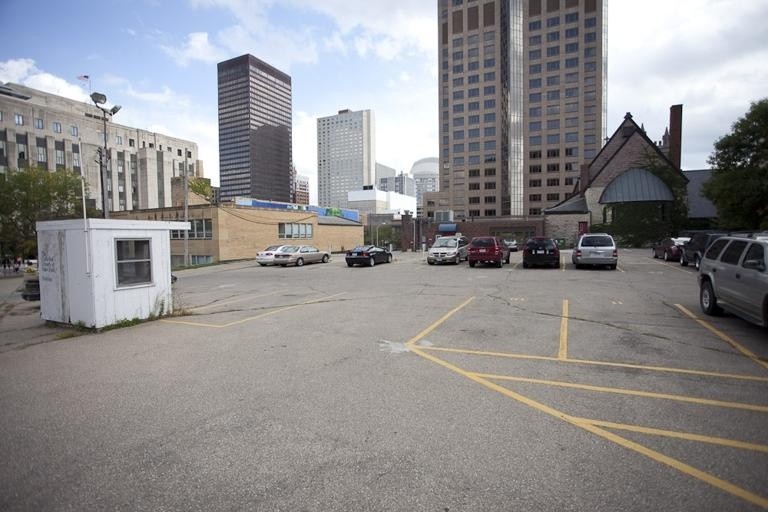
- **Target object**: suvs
[696,237,768,332]
[427,236,518,268]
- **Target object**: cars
[255,244,331,266]
[345,245,393,267]
[652,231,768,272]
[523,236,561,269]
[571,233,618,271]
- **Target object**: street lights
[376,223,387,248]
[90,92,122,219]
